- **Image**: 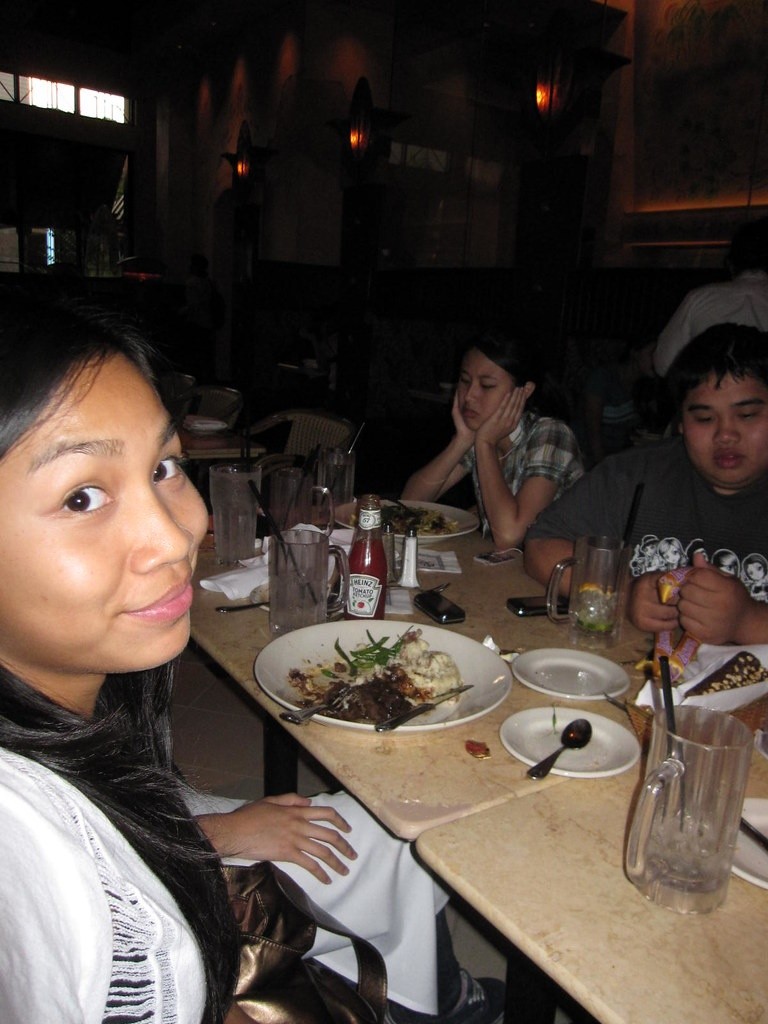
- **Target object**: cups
[272,468,334,533]
[210,463,262,565]
[624,705,754,914]
[317,448,355,508]
[268,530,350,636]
[546,537,632,647]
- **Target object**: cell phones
[506,595,570,617]
[414,591,465,624]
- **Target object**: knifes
[374,684,475,733]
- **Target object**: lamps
[220,119,281,203]
[472,7,632,161]
[325,75,413,188]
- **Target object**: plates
[729,796,768,888]
[511,648,630,700]
[254,619,511,734]
[334,498,479,543]
[499,706,640,779]
[250,584,269,611]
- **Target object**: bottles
[380,522,396,585]
[397,526,420,588]
[343,494,388,619]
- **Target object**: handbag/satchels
[215,860,386,1024]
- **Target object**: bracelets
[418,473,447,484]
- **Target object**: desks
[187,532,768,1024]
[174,425,268,458]
[406,387,454,404]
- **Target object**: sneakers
[389,968,505,1024]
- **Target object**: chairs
[153,372,355,518]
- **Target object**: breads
[685,649,768,701]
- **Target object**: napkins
[200,524,354,601]
[383,585,413,615]
[393,543,463,574]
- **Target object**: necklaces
[497,428,526,462]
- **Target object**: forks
[277,684,355,725]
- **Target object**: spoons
[526,719,593,780]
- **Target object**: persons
[523,322,768,646]
[649,217,768,389]
[0,274,556,1024]
[400,329,588,551]
[528,337,698,447]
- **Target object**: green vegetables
[318,625,418,678]
[382,505,430,523]
